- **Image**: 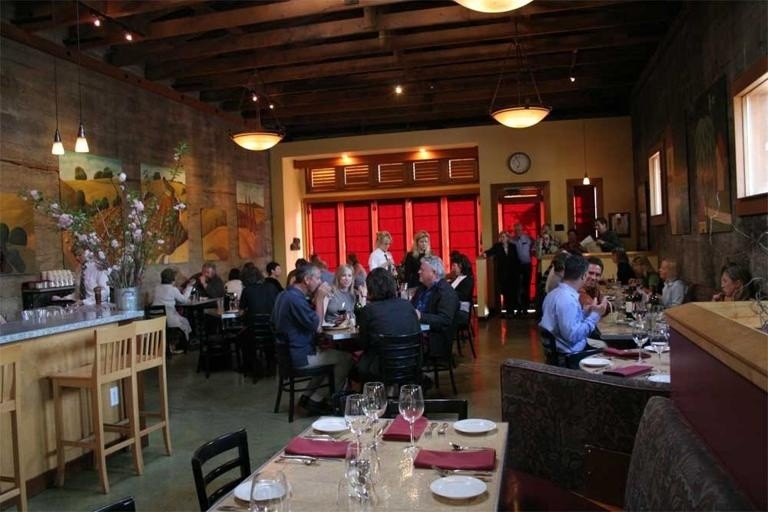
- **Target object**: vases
[114,287,139,310]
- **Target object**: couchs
[505,396,753,511]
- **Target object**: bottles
[42,269,75,287]
[650,283,660,313]
[354,293,363,315]
[625,287,634,316]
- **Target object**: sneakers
[304,397,334,415]
[298,395,311,407]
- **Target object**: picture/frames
[608,212,632,238]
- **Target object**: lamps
[73,2,89,154]
[582,119,591,186]
[49,3,66,157]
[225,69,289,151]
[487,15,554,129]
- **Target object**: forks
[304,434,347,440]
[432,464,494,476]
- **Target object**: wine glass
[338,298,348,325]
[250,471,293,512]
[398,384,425,460]
[633,313,670,373]
[360,381,388,473]
[345,393,374,459]
[345,442,382,505]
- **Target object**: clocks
[508,152,531,174]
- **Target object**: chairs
[89,496,139,512]
[197,313,241,378]
[272,332,336,422]
[426,302,461,395]
[145,305,180,360]
[191,425,252,512]
[370,332,424,400]
[538,325,564,368]
[457,301,478,359]
[237,313,276,384]
[363,399,468,420]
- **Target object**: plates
[429,475,488,500]
[583,354,612,368]
[311,417,352,433]
[234,479,286,503]
[326,325,350,331]
[453,419,496,435]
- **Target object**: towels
[604,347,651,360]
[381,413,429,442]
[284,436,363,459]
[413,448,496,470]
[604,365,653,379]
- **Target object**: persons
[482,216,686,368]
[710,260,758,302]
[70,238,111,308]
[146,230,476,417]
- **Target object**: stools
[0,343,28,512]
[48,320,145,493]
[99,316,173,473]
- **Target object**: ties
[80,263,86,300]
[384,253,391,272]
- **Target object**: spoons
[424,423,437,435]
[219,504,268,512]
[438,422,449,435]
[274,454,318,466]
[452,443,490,452]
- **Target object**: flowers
[15,139,192,288]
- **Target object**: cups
[20,306,78,318]
[338,479,378,512]
